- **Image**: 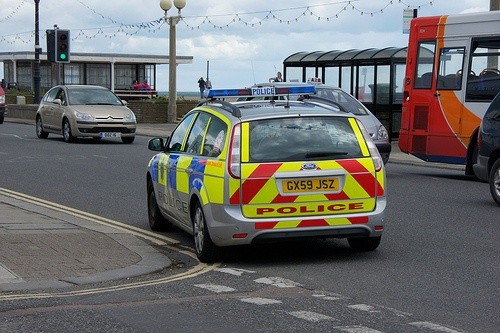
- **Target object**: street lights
[160,0,187,123]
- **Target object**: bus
[398,9,500,183]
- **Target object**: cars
[35,84,137,144]
[145,84,392,263]
[472,90,500,206]
[0,82,7,124]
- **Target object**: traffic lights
[55,29,71,63]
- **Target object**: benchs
[113,89,158,99]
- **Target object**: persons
[132,80,150,89]
[274,72,282,82]
[197,77,206,99]
[205,78,212,89]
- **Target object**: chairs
[421,68,500,93]
